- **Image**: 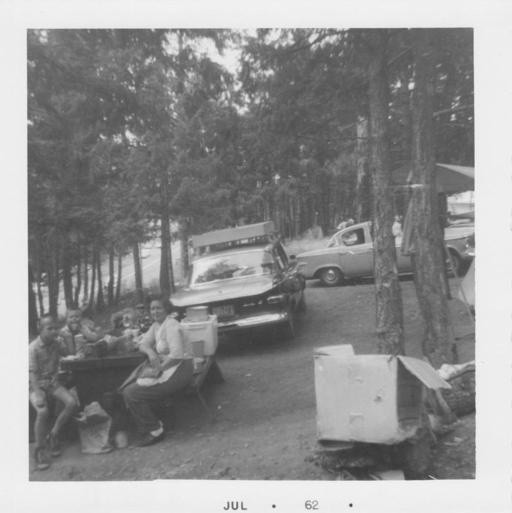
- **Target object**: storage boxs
[179,314,220,355]
[309,342,456,446]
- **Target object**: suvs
[168,239,307,345]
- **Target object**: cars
[297,218,475,284]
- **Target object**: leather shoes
[140,433,164,447]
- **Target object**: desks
[55,349,149,431]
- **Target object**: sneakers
[35,447,49,470]
[47,433,62,455]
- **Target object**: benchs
[171,353,225,420]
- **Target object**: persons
[392,215,403,246]
[336,218,354,230]
[342,231,358,246]
[59,308,103,357]
[29,313,77,470]
[122,293,195,448]
